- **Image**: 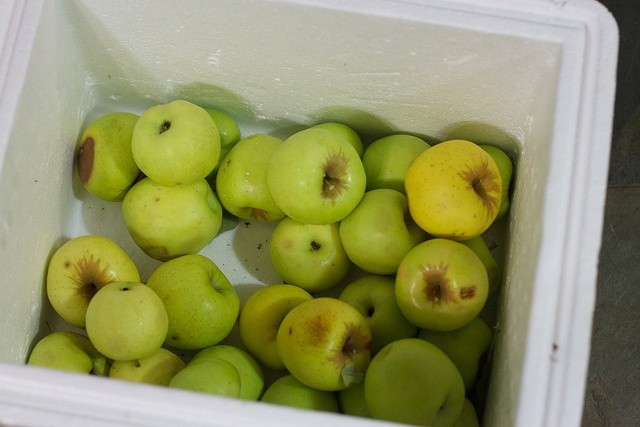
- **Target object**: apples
[269,216,352,294]
[238,284,315,370]
[476,145,513,223]
[216,134,286,224]
[464,235,501,298]
[122,177,223,263]
[338,384,369,418]
[258,375,340,414]
[77,112,142,203]
[167,357,242,400]
[131,99,221,187]
[404,139,503,242]
[394,238,490,333]
[310,122,365,160]
[363,338,465,427]
[338,278,419,356]
[145,253,240,351]
[46,235,141,330]
[192,345,265,401]
[419,317,493,395]
[458,399,480,427]
[108,348,186,386]
[202,108,241,170]
[276,297,373,392]
[85,280,169,362]
[339,188,426,276]
[266,127,367,225]
[26,330,108,379]
[362,134,432,193]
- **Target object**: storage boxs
[0,0,620,426]
